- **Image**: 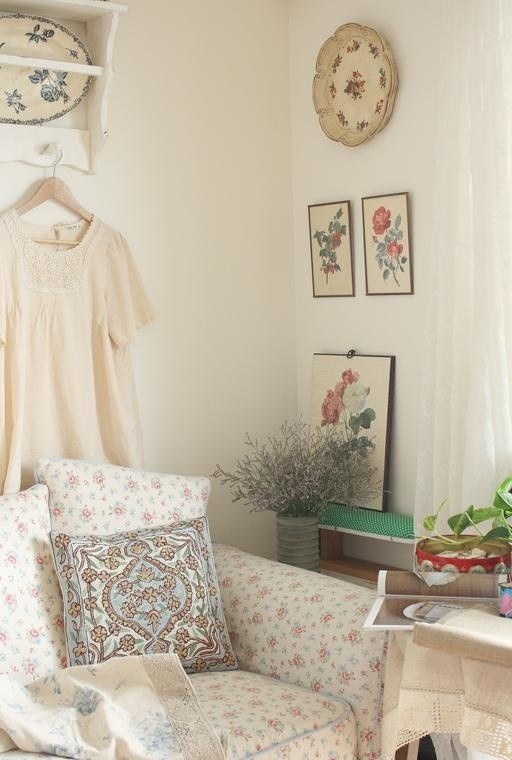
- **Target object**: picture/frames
[307,192,415,298]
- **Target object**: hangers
[1,140,123,249]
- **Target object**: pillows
[48,516,240,674]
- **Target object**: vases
[276,514,321,573]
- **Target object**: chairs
[2,457,392,760]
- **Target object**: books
[362,570,507,630]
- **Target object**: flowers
[208,414,393,519]
